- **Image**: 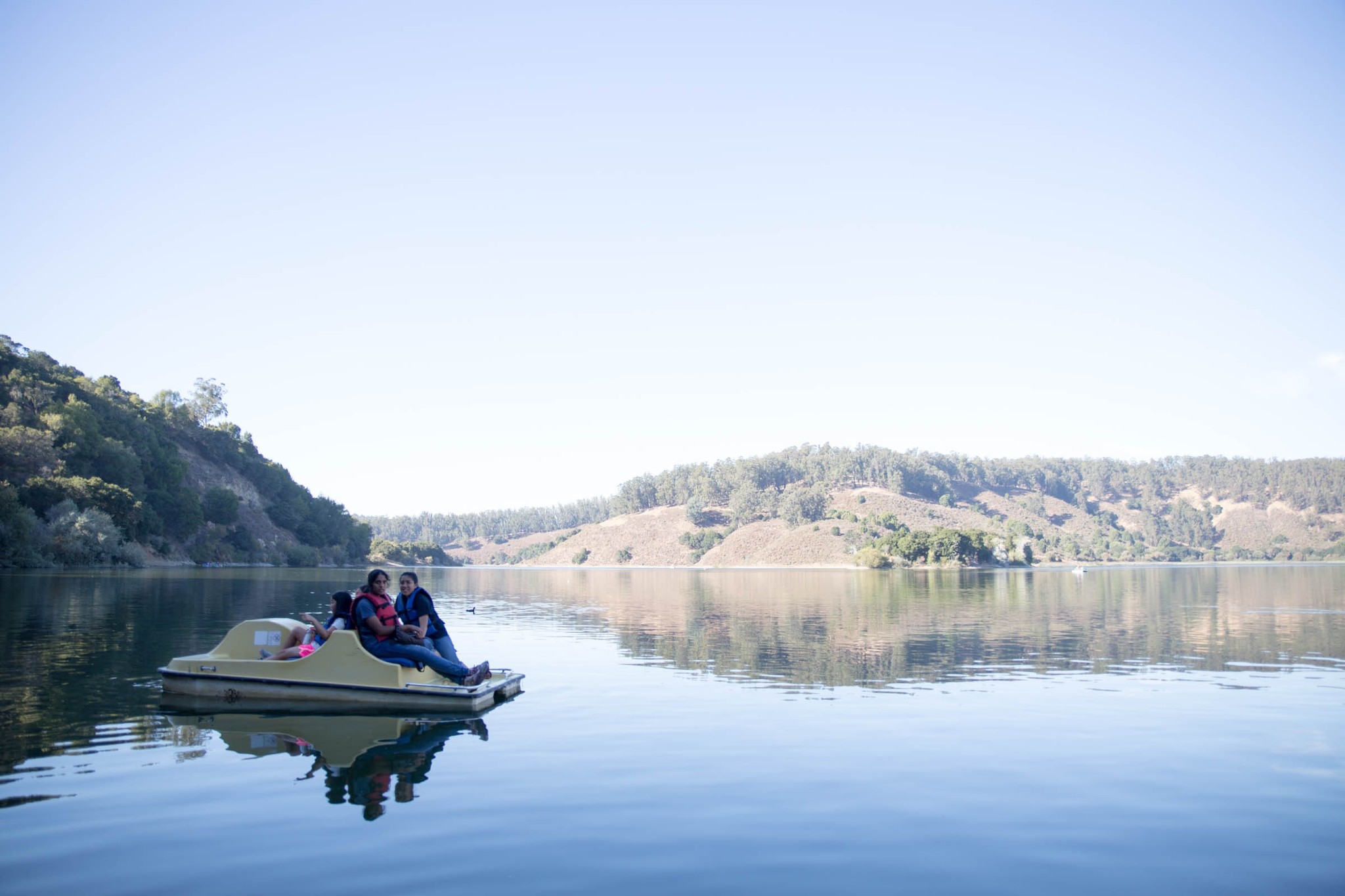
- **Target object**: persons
[274,718,488,822]
[351,569,489,692]
[257,585,367,660]
[394,571,492,679]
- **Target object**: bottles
[302,626,317,644]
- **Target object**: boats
[201,561,225,568]
[153,616,526,723]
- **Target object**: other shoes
[485,671,491,679]
[258,649,273,658]
[468,660,489,677]
[464,665,488,693]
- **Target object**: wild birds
[467,607,476,612]
